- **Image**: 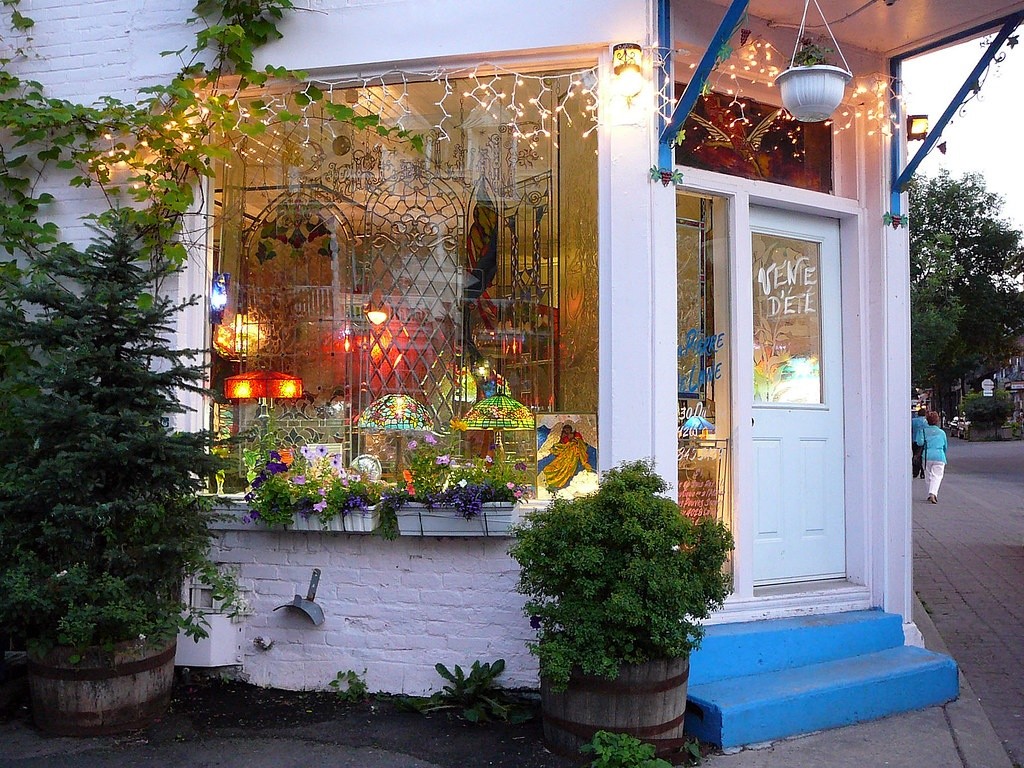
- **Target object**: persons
[916,411,948,504]
[912,408,928,479]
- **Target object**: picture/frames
[533,411,600,500]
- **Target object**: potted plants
[0,413,255,735]
[774,33,853,123]
[510,458,735,739]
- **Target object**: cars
[949,417,971,439]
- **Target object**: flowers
[375,434,533,542]
[243,442,379,529]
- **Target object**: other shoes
[913,472,925,479]
[928,496,937,504]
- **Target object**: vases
[398,500,523,538]
[286,501,376,532]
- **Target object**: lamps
[226,366,302,430]
[365,289,389,325]
[907,113,927,142]
[360,395,434,476]
[211,272,230,322]
[461,387,536,466]
[611,44,645,99]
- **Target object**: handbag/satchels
[913,445,925,466]
